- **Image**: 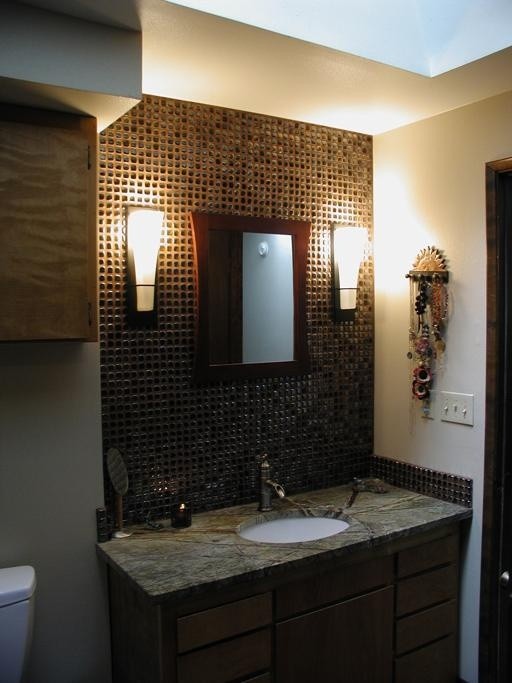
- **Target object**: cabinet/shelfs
[108,568,274,683]
[396,523,460,683]
[0,104,98,342]
[277,543,395,682]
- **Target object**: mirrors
[190,211,311,385]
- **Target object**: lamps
[125,206,166,324]
[331,221,369,323]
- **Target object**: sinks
[235,506,350,543]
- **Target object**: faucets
[256,452,287,513]
